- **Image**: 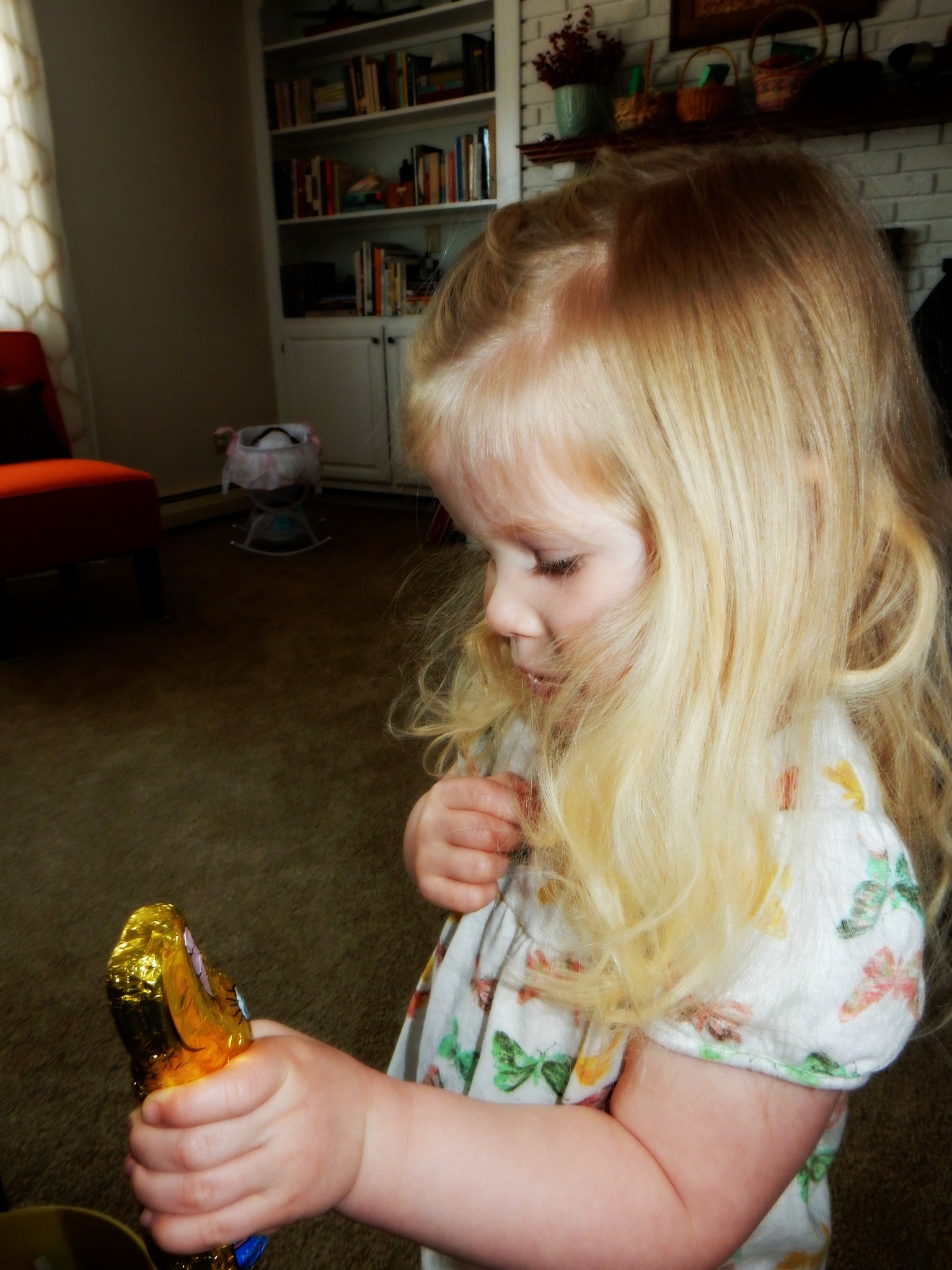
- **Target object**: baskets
[747,6,827,113]
[613,41,674,131]
[676,46,738,123]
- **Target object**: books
[279,240,440,319]
[272,26,495,221]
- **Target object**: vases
[553,85,609,138]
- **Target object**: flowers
[531,4,625,90]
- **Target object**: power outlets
[213,431,229,453]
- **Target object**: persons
[126,140,952,1270]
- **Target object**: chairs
[0,331,167,617]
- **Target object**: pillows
[0,376,73,465]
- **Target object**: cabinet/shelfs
[270,317,437,497]
[244,0,520,317]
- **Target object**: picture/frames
[669,0,875,52]
